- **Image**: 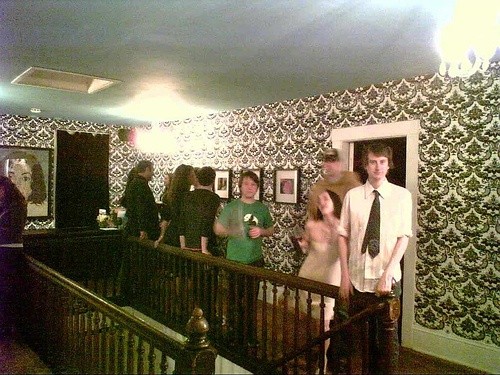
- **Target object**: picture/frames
[240,169,262,201]
[212,169,232,200]
[273,169,300,203]
[0,145,52,219]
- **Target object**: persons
[0,175,27,345]
[155,164,226,327]
[0,151,48,216]
[215,170,274,347]
[116,160,160,296]
[326,143,414,375]
[307,148,361,219]
[293,190,343,375]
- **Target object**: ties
[361,190,380,258]
